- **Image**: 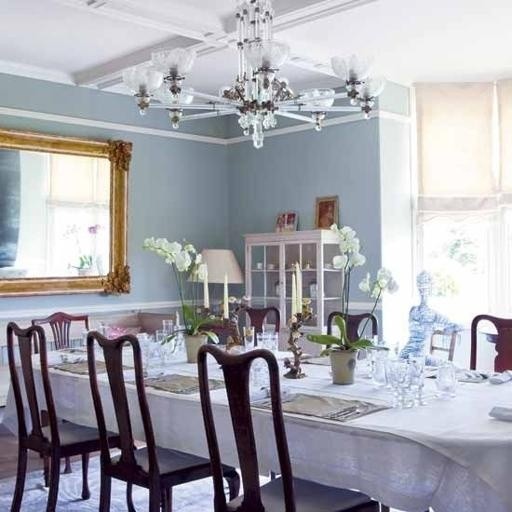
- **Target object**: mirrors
[1,127,133,298]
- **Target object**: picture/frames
[274,211,298,232]
[314,195,340,229]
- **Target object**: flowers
[307,223,399,357]
[75,223,99,268]
[143,236,219,344]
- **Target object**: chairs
[196,344,389,512]
[6,321,137,512]
[471,315,512,372]
[31,311,90,474]
[429,329,456,361]
[224,301,281,346]
[86,331,240,512]
[326,311,378,357]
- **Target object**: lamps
[121,0,389,149]
[185,247,244,284]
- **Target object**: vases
[329,351,358,385]
[183,335,208,363]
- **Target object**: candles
[222,273,230,318]
[292,261,302,323]
[204,266,210,311]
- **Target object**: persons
[280,215,285,232]
[320,205,334,228]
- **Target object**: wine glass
[363,333,388,377]
[385,357,429,412]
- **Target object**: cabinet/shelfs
[243,229,344,355]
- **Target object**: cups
[243,326,255,351]
[436,360,458,399]
[136,318,185,377]
[251,330,282,387]
[371,353,388,391]
